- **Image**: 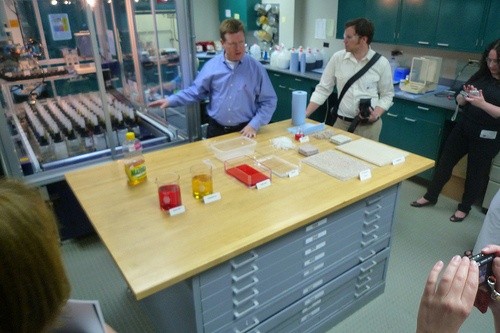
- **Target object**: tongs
[338,136,409,166]
[302,149,375,181]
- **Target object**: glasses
[486,57,498,64]
[227,41,245,48]
[343,33,356,41]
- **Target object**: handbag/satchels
[309,82,339,127]
[439,102,460,143]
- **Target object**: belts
[337,114,353,123]
[209,119,243,133]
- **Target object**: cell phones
[469,90,480,98]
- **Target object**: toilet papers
[290,52,299,72]
[291,90,308,126]
[300,53,306,72]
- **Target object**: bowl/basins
[212,134,259,162]
[221,155,273,188]
[252,144,302,178]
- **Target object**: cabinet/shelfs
[337,0,500,54]
[219,0,261,31]
[146,185,401,333]
[267,69,451,186]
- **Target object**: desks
[61,118,436,333]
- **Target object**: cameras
[468,252,497,313]
[359,98,370,118]
[460,90,469,97]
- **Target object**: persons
[0,177,116,333]
[149,19,278,138]
[306,18,394,142]
[409,38,500,222]
[416,245,500,333]
[464,189,500,258]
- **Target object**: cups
[188,162,213,200]
[156,170,182,211]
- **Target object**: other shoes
[410,196,434,208]
[449,209,469,223]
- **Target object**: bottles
[250,43,261,61]
[22,87,143,162]
[262,41,270,61]
[270,41,324,74]
[122,131,147,185]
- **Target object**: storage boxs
[209,135,257,160]
[402,55,443,94]
[224,155,271,189]
[254,150,301,178]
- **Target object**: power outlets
[468,59,480,67]
